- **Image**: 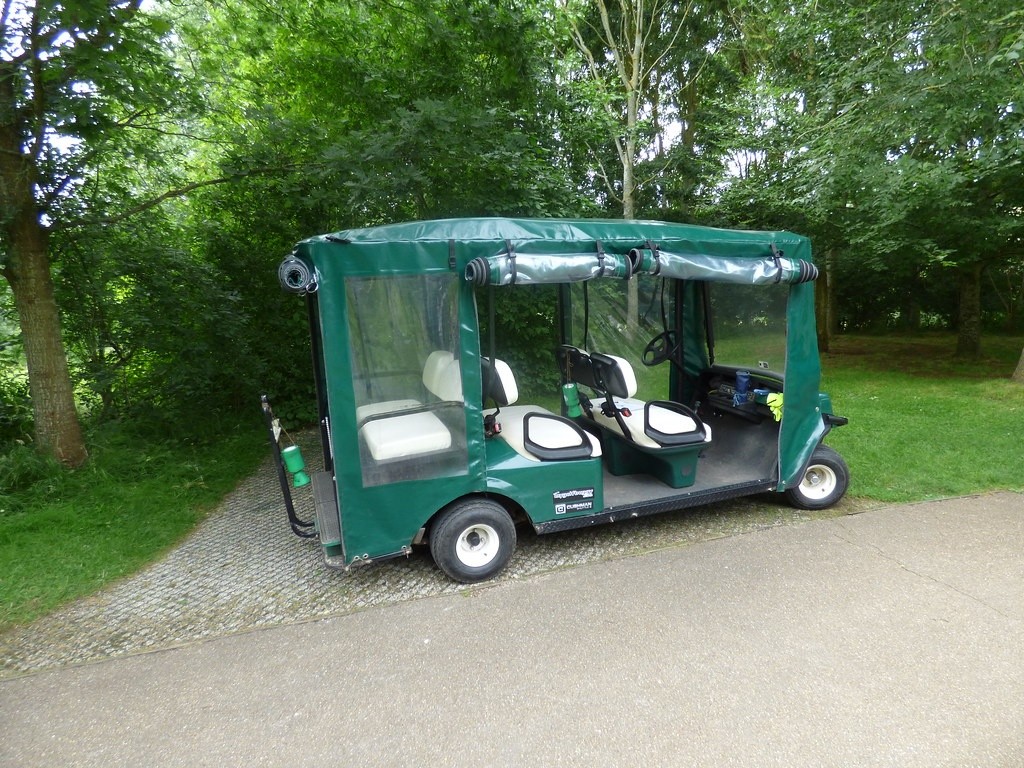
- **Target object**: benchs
[354,349,460,469]
[555,344,713,452]
[466,350,603,460]
